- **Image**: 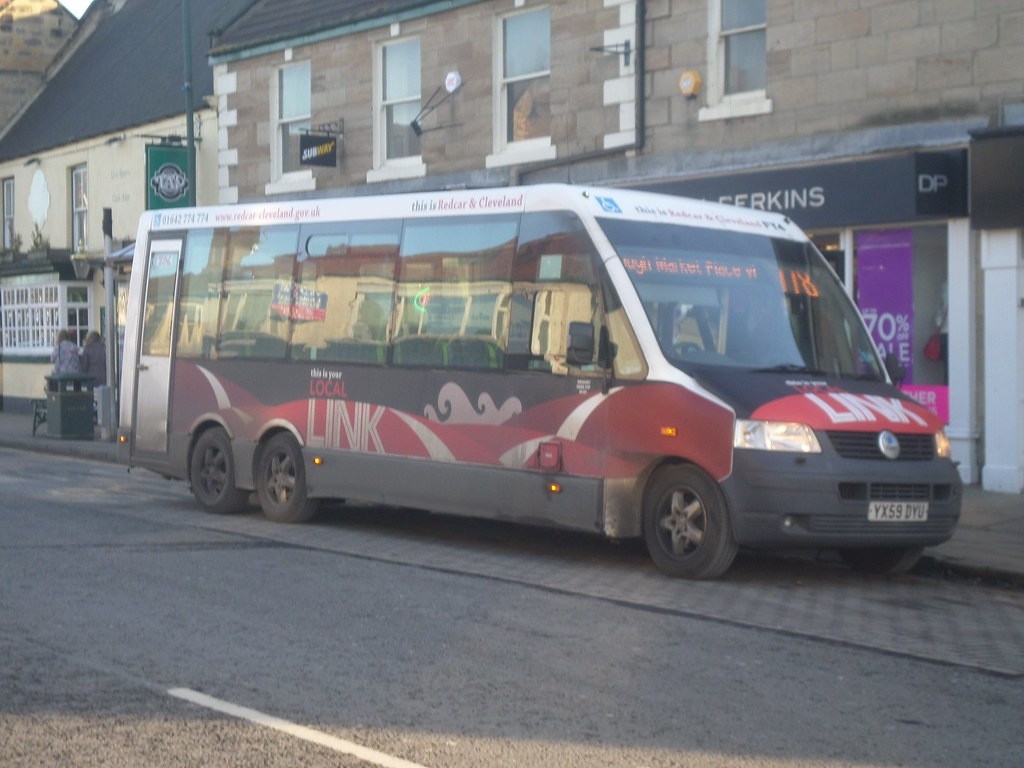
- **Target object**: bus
[112,180,966,583]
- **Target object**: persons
[51,331,80,377]
[80,332,107,387]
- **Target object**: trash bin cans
[44,371,95,439]
